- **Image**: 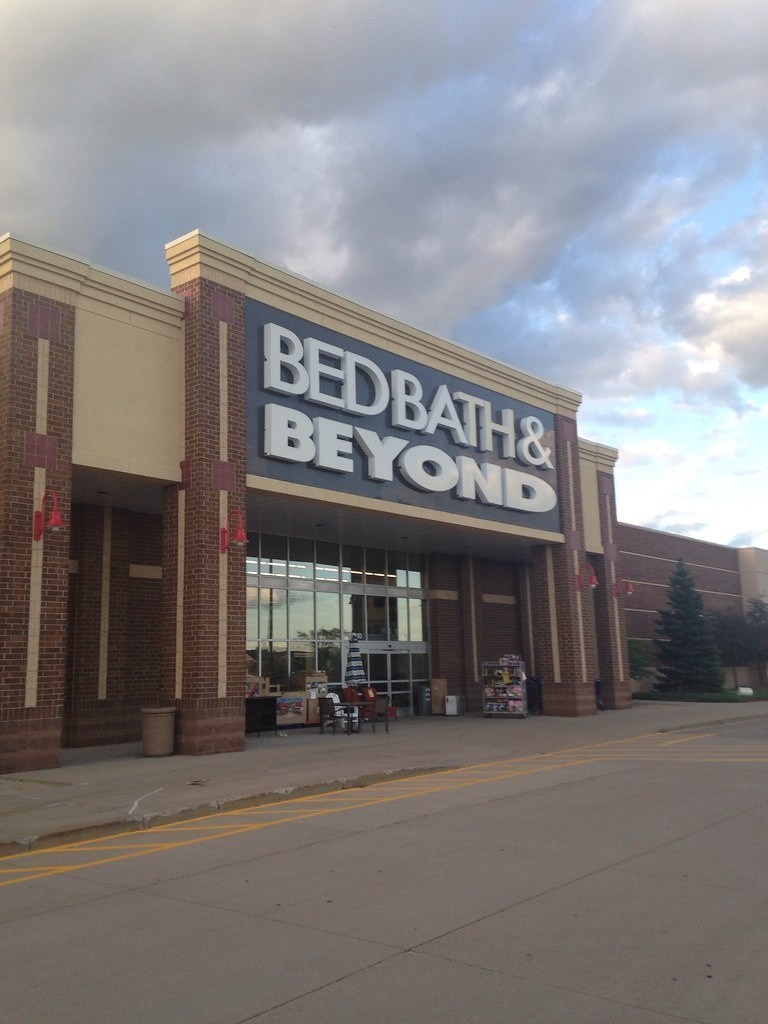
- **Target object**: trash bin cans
[140,706,178,757]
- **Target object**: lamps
[34,491,65,541]
[221,509,248,553]
[576,562,599,591]
[612,572,635,599]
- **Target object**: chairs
[318,698,347,736]
[361,694,390,734]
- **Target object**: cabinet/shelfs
[480,662,528,718]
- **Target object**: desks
[333,702,372,735]
[245,696,281,738]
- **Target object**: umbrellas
[345,633,367,734]
